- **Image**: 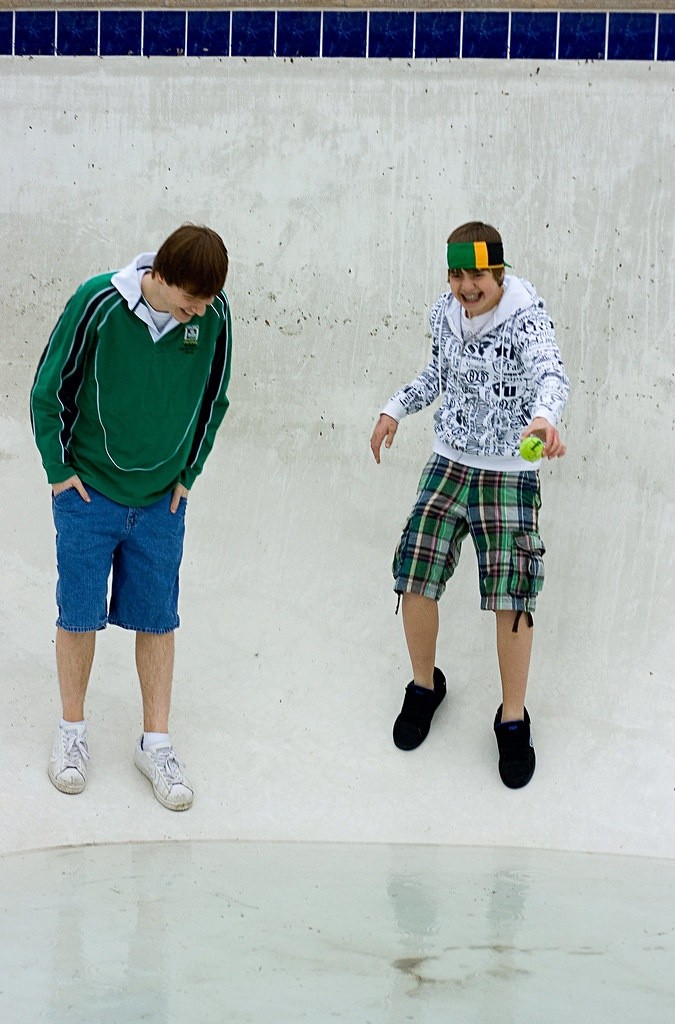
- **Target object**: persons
[31,221,232,810]
[371,222,569,789]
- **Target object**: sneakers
[49,724,91,793]
[135,735,194,810]
[392,667,447,751]
[494,702,535,789]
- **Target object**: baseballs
[520,437,544,461]
